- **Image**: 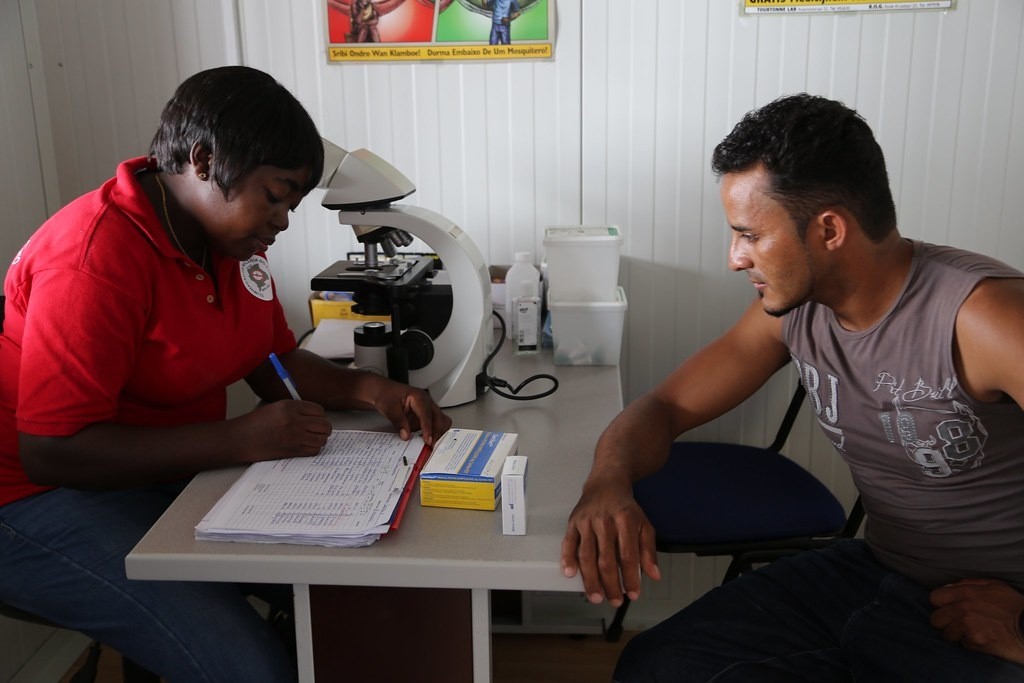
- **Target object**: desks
[125,328,639,683]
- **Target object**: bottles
[505,250,541,356]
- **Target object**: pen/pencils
[269,353,302,401]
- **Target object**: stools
[0,606,102,683]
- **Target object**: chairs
[599,373,868,646]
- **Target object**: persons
[559,91,1024,683]
[0,65,453,683]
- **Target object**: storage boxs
[307,291,392,328]
[488,224,628,366]
[500,456,529,536]
[420,428,519,512]
